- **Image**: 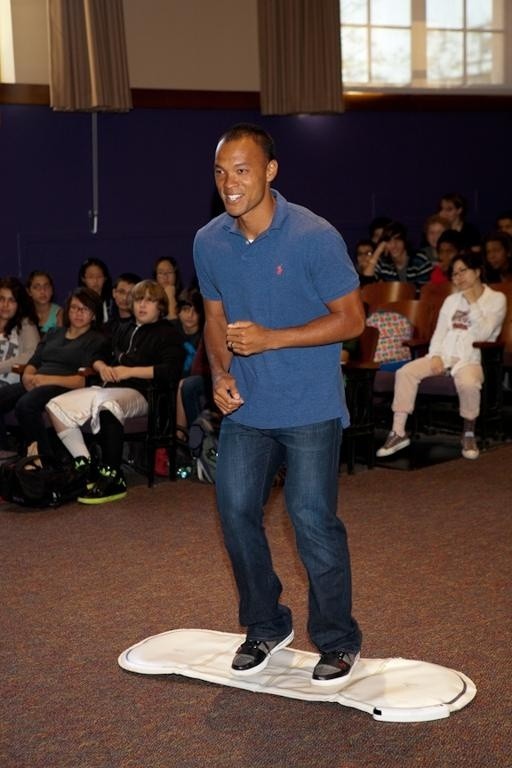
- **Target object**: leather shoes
[150,464,170,487]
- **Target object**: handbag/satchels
[183,421,221,485]
[1,454,71,511]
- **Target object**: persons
[1,258,211,506]
[375,256,507,461]
[340,339,360,387]
[192,127,365,685]
[357,195,510,283]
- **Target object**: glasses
[69,304,90,314]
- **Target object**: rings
[229,341,234,348]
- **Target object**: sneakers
[76,466,129,504]
[376,431,411,457]
[459,433,480,460]
[311,650,361,686]
[231,630,295,677]
[75,456,102,490]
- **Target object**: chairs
[0,279,512,488]
